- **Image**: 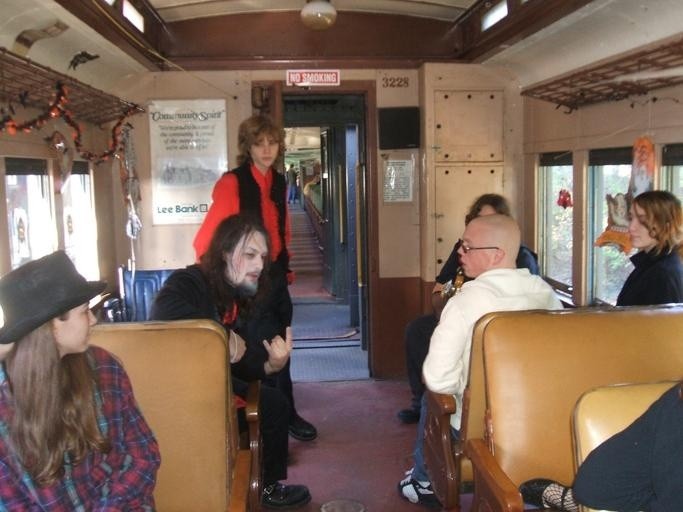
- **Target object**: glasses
[457,238,499,254]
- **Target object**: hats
[0,250,106,344]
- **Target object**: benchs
[464,308,682,512]
[414,309,503,510]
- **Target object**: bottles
[103,297,122,322]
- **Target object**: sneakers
[398,476,439,505]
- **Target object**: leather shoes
[260,480,311,509]
[398,406,421,423]
[287,413,316,440]
[518,478,578,512]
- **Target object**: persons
[395,191,541,427]
[146,208,313,507]
[612,187,682,305]
[394,210,562,505]
[515,374,683,509]
[286,163,296,204]
[0,250,164,508]
[188,114,317,439]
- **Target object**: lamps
[299,0,337,31]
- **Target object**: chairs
[103,267,178,321]
